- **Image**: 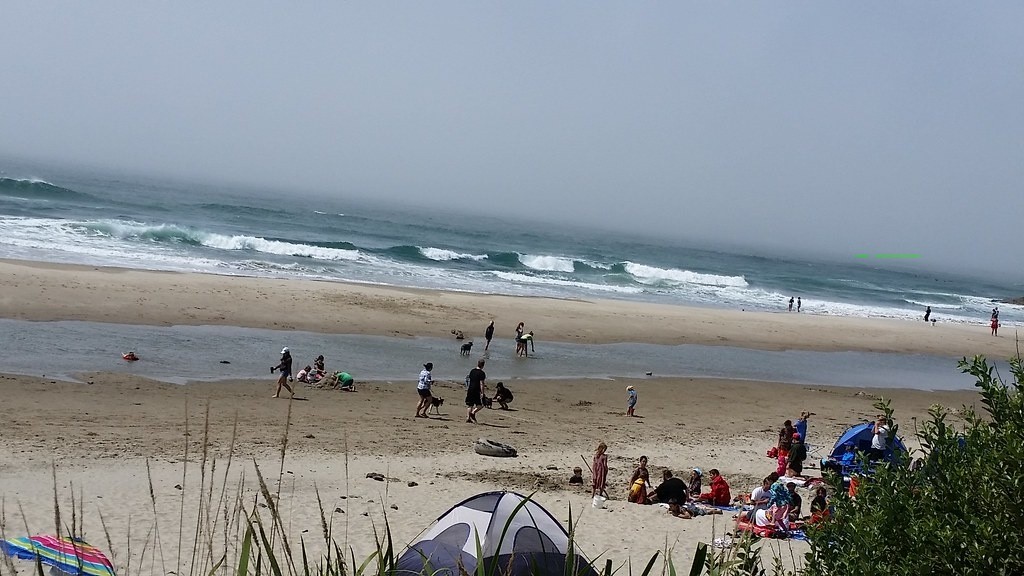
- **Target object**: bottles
[271,367,273,373]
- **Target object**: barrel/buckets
[592,495,606,509]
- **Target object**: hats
[844,441,855,447]
[129,352,134,355]
[495,382,503,388]
[792,433,800,439]
[280,347,290,354]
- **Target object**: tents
[385,492,604,576]
[829,421,908,472]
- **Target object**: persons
[569,442,732,519]
[732,471,835,534]
[123,351,137,358]
[626,386,637,416]
[925,306,931,322]
[414,363,433,417]
[990,307,1000,336]
[271,347,356,397]
[789,297,801,312]
[776,411,890,488]
[486,321,494,350]
[465,359,485,423]
[493,382,513,409]
[515,321,535,357]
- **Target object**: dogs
[460,341,473,355]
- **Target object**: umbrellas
[0,536,115,576]
